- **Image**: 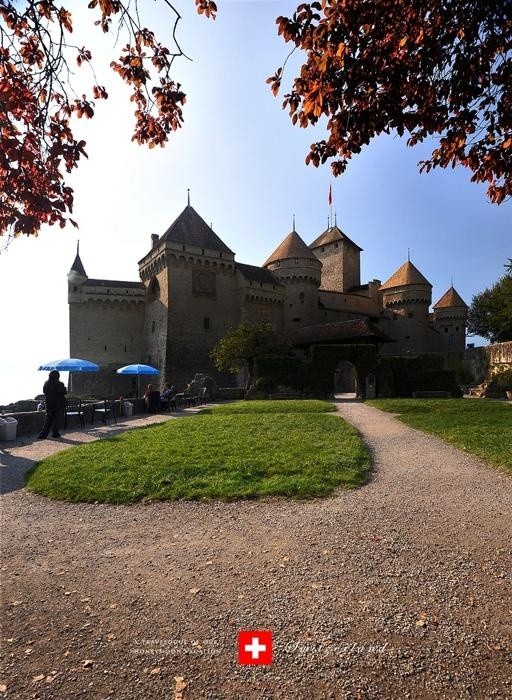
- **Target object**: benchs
[269,392,306,400]
[412,391,452,398]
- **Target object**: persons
[143,382,154,403]
[37,401,45,410]
[185,382,197,406]
[160,382,174,403]
[35,369,68,439]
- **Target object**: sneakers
[37,432,62,438]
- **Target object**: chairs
[64,388,206,429]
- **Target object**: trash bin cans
[124,401,134,417]
[0,417,18,441]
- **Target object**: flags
[329,184,332,204]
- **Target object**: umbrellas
[115,363,160,398]
[37,358,99,391]
